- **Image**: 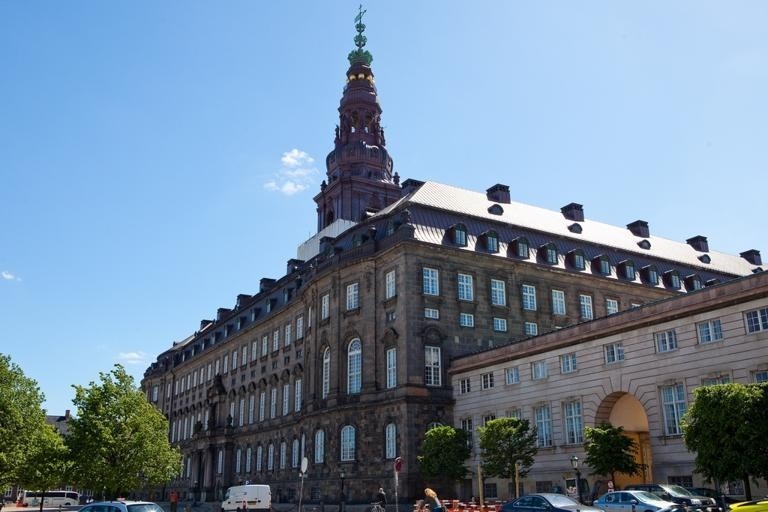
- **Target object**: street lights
[570,455,579,500]
[192,480,198,505]
[339,467,346,512]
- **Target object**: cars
[499,493,604,512]
[594,483,731,512]
[77,500,164,512]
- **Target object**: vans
[221,484,272,512]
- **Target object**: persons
[376,487,387,508]
[418,488,444,511]
[0,492,5,512]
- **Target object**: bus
[23,490,80,507]
